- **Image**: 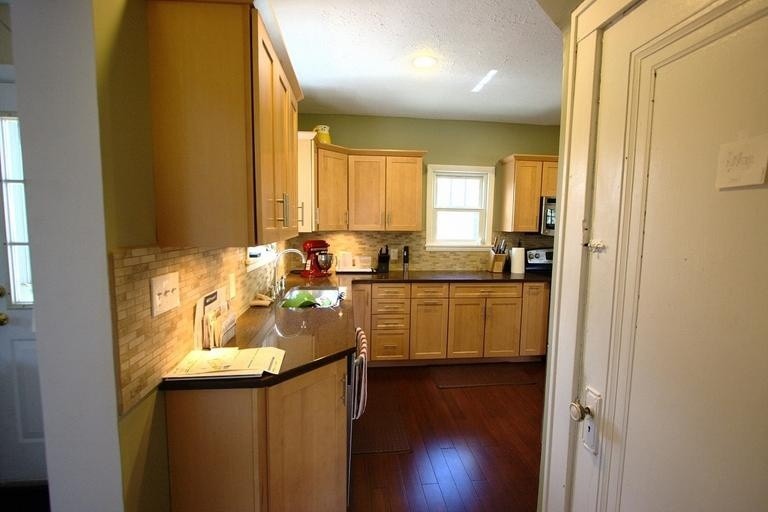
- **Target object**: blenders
[301,239,334,277]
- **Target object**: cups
[377,254,390,273]
[337,251,372,270]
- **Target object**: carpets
[428,362,537,388]
[352,395,414,455]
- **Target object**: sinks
[274,285,341,310]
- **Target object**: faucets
[271,248,306,297]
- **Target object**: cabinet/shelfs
[371,283,449,368]
[148,0,289,248]
[298,131,348,233]
[500,153,543,233]
[289,83,305,240]
[520,282,550,365]
[164,354,353,512]
[540,155,559,197]
[447,282,523,365]
[352,283,372,367]
[348,147,428,232]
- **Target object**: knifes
[493,236,507,254]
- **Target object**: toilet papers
[510,247,526,274]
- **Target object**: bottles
[403,246,409,272]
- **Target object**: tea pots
[312,125,331,145]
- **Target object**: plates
[335,267,373,274]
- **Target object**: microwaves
[540,197,557,237]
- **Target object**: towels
[249,294,274,308]
[351,327,368,421]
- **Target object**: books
[162,346,286,382]
